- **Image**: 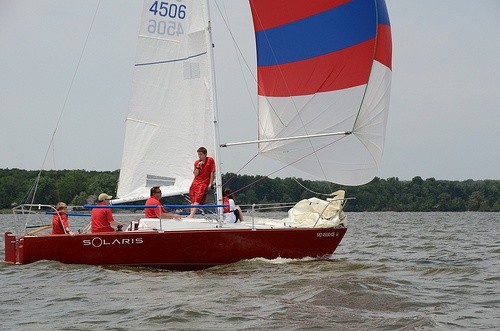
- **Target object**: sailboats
[3,0,393,273]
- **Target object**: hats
[98,193,113,201]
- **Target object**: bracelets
[196,166,200,169]
[68,230,71,232]
[210,182,212,184]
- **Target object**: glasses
[155,191,161,193]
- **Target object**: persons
[52,202,75,237]
[91,193,123,232]
[145,186,182,219]
[222,188,244,223]
[187,147,215,218]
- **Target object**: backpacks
[223,197,230,213]
